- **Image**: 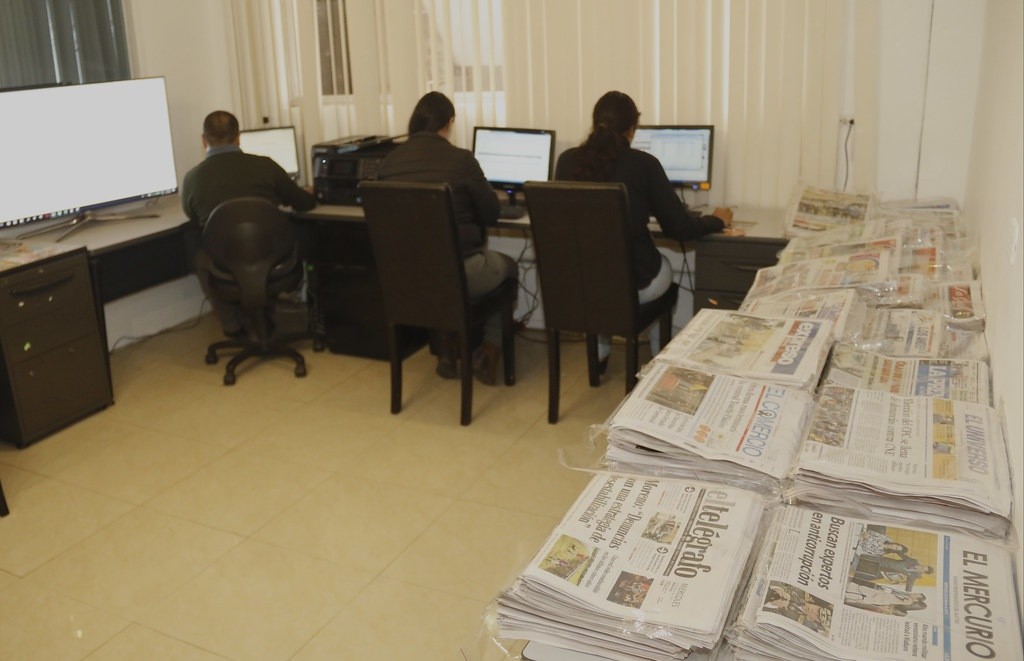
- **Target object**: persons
[375,89,520,384]
[554,90,734,379]
[180,110,315,340]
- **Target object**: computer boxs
[312,135,375,204]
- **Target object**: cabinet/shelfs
[1,240,116,450]
[693,215,791,317]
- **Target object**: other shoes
[434,354,461,380]
[473,341,500,387]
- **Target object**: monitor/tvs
[471,126,556,190]
[629,124,716,190]
[240,126,300,178]
[1,76,180,228]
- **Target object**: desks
[22,197,663,305]
[520,640,616,661]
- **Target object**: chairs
[356,180,518,427]
[522,179,679,424]
[204,195,306,386]
